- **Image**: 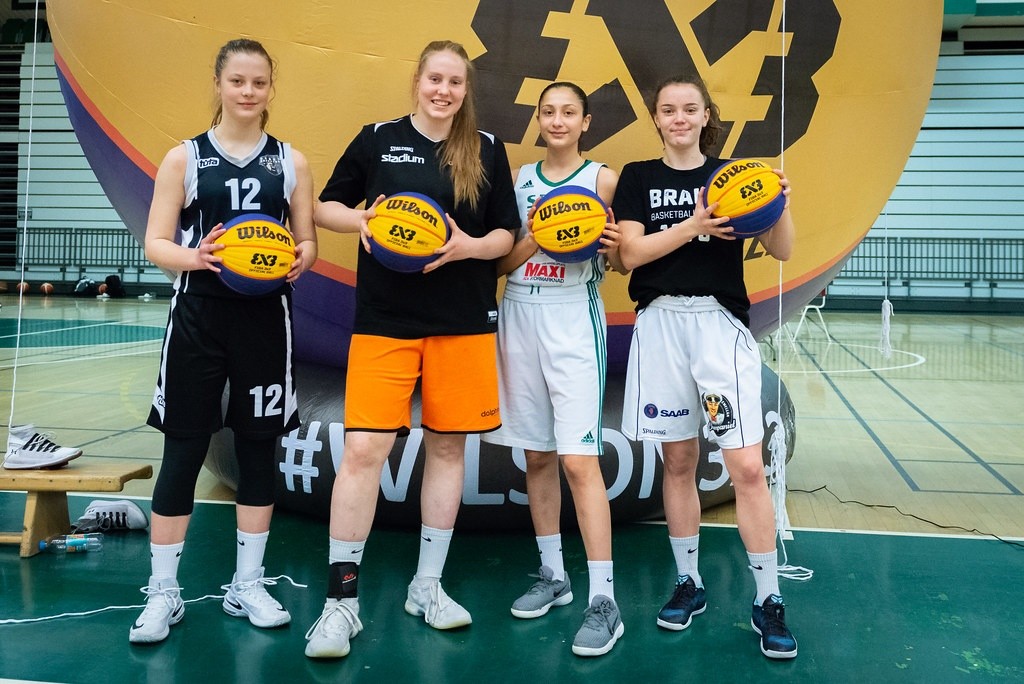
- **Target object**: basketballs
[531,185,610,264]
[702,157,786,239]
[211,213,295,297]
[367,191,448,272]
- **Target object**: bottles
[39,533,105,552]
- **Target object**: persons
[496,83,629,657]
[304,40,521,658]
[128,39,318,642]
[612,75,798,659]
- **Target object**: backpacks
[72,275,127,299]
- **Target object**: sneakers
[404,574,472,628]
[573,595,625,656]
[305,596,364,657]
[128,575,183,642]
[510,566,574,618]
[656,575,707,630]
[751,593,798,658]
[221,567,291,628]
[4,423,83,469]
[78,499,148,533]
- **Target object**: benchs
[0,461,153,556]
